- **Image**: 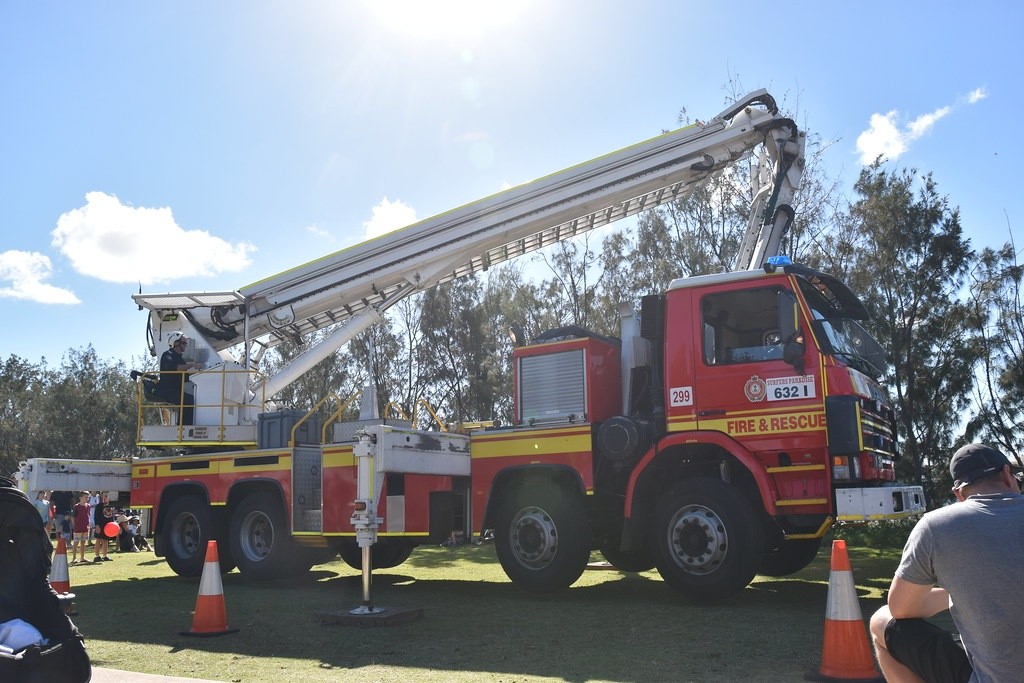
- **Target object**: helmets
[168,331,184,349]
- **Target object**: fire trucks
[129,88,934,611]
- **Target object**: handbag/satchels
[0,619,49,660]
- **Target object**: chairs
[142,379,179,424]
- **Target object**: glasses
[180,342,187,345]
[133,516,138,518]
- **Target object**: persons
[870,443,1024,683]
[159,331,204,425]
[33,491,155,564]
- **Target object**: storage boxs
[256,410,321,450]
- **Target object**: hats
[132,516,139,520]
[950,444,1020,490]
[117,515,132,525]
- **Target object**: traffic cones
[45,538,79,615]
[176,531,242,637]
[793,533,891,683]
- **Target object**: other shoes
[88,540,94,546]
[80,558,90,561]
[147,546,154,552]
[67,543,73,548]
[71,559,77,563]
[102,556,113,561]
[94,557,104,562]
[133,547,140,553]
